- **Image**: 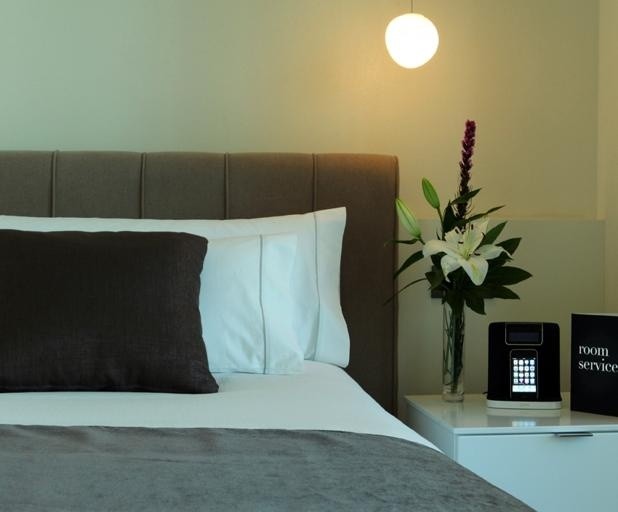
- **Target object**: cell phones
[509,349,539,401]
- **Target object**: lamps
[381,1,440,72]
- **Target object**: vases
[439,301,467,403]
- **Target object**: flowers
[382,120,532,394]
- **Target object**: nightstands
[402,394,617,511]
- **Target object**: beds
[1,150,536,512]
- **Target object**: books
[569,311,618,419]
